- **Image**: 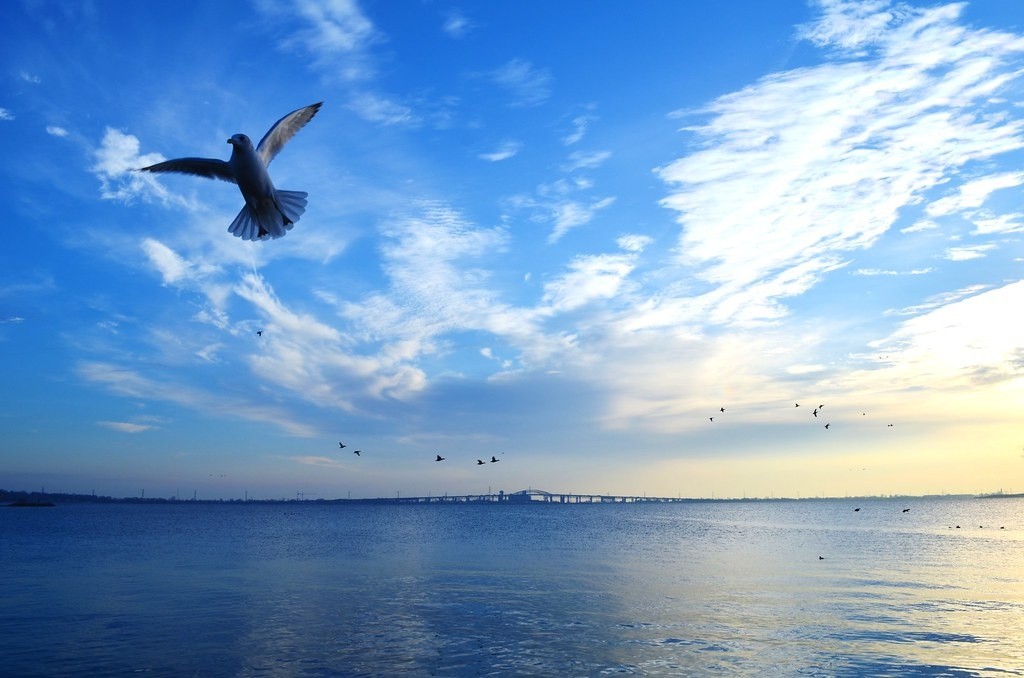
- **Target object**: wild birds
[339,442,347,449]
[709,417,714,421]
[354,451,361,456]
[813,409,818,417]
[477,459,485,465]
[824,423,830,429]
[435,455,445,461]
[795,404,800,408]
[720,408,725,412]
[818,405,824,409]
[490,456,500,463]
[257,330,263,336]
[816,354,913,512]
[136,101,326,240]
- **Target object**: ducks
[949,522,1007,531]
[819,555,824,560]
